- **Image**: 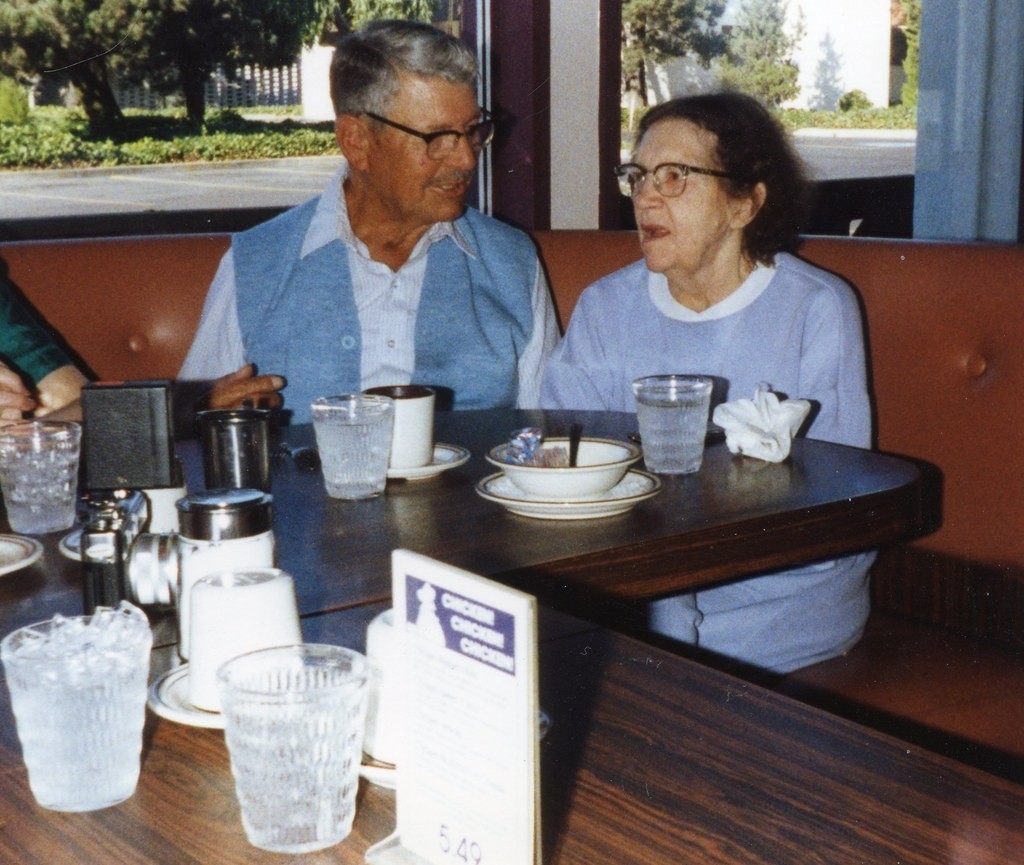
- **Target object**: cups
[0,614,153,813]
[1,419,82,534]
[362,608,395,766]
[189,566,302,715]
[362,385,436,468]
[215,643,373,851]
[634,376,714,474]
[309,393,393,500]
[194,408,277,490]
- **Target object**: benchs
[0,228,1023,741]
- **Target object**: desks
[0,573,1023,865]
[0,398,945,672]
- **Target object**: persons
[534,92,879,678]
[0,259,92,451]
[171,20,562,440]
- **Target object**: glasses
[356,108,496,160]
[613,162,743,198]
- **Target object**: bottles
[125,487,189,611]
[174,490,277,662]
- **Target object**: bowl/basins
[485,435,643,496]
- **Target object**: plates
[358,706,548,790]
[149,643,368,728]
[0,536,43,575]
[386,442,471,479]
[58,527,82,562]
[473,469,665,518]
